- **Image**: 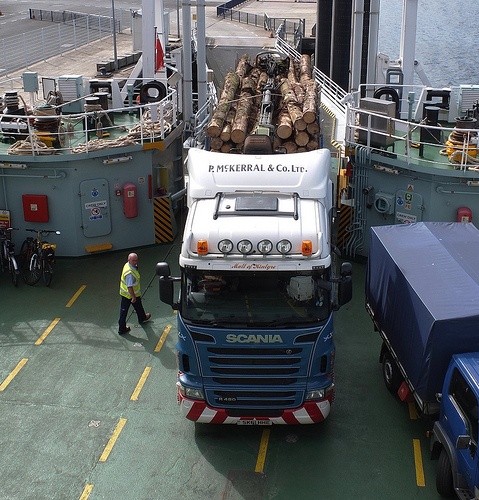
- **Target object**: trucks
[365,220,479,500]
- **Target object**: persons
[118,253,151,335]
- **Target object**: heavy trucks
[155,92,355,425]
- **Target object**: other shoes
[120,326,130,334]
[145,313,151,321]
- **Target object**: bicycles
[19,228,63,288]
[0,227,23,287]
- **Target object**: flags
[154,30,164,72]
